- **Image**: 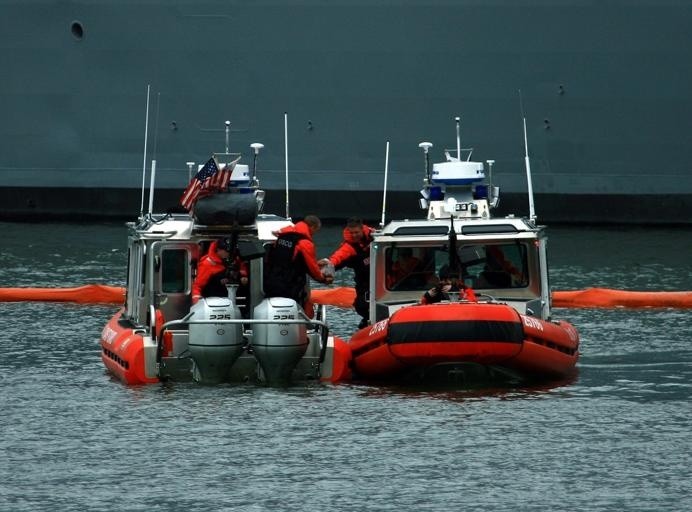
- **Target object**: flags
[179,158,240,210]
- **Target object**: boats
[99,85,352,387]
[345,88,580,382]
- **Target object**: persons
[263,215,377,321]
[192,239,249,304]
[421,275,479,305]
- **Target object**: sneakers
[358,318,367,328]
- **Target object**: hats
[217,239,230,252]
